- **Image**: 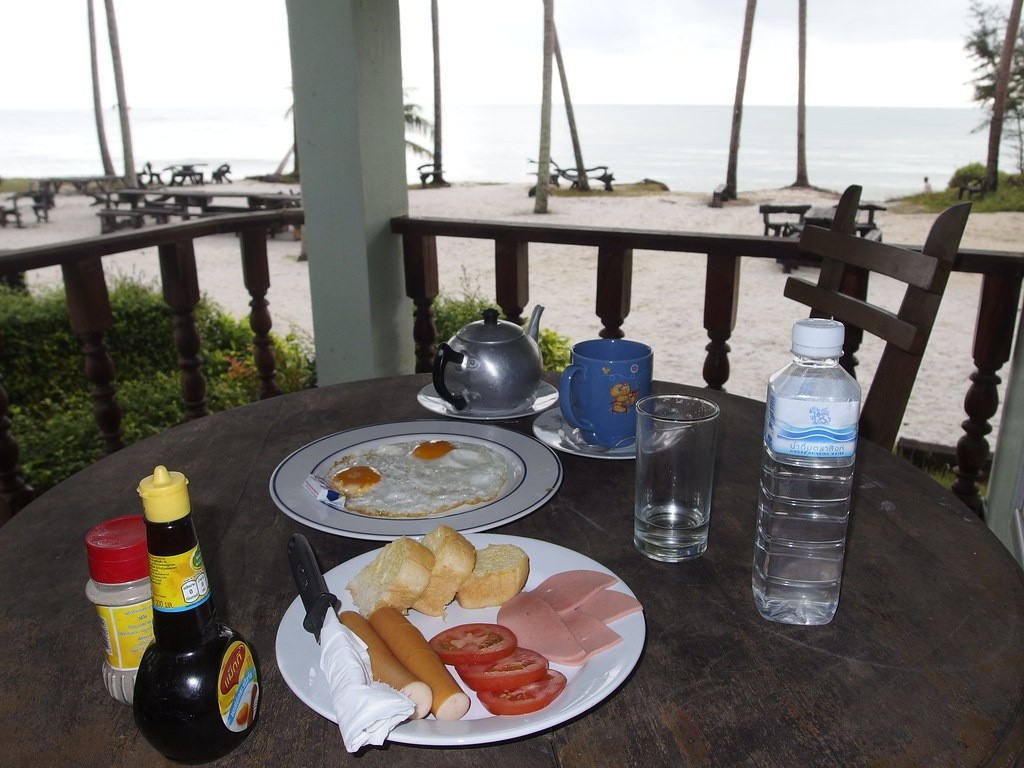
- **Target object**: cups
[558,339,654,449]
[633,393,721,563]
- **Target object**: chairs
[782,185,974,454]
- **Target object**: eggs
[380,436,508,503]
[324,446,468,517]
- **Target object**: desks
[0,372,1024,768]
[172,163,210,186]
[800,204,862,227]
[114,187,300,238]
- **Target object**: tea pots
[431,304,545,417]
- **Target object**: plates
[269,420,564,542]
[274,532,646,747]
[532,407,636,460]
[416,378,560,420]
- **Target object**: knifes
[285,534,338,646]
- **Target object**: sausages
[338,606,470,720]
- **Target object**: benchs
[756,204,886,274]
[0,173,303,240]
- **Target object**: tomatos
[427,623,568,715]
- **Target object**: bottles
[135,465,263,767]
[749,318,862,627]
[82,515,156,707]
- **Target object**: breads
[346,534,434,619]
[457,543,529,608]
[410,524,476,616]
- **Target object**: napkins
[316,605,416,753]
